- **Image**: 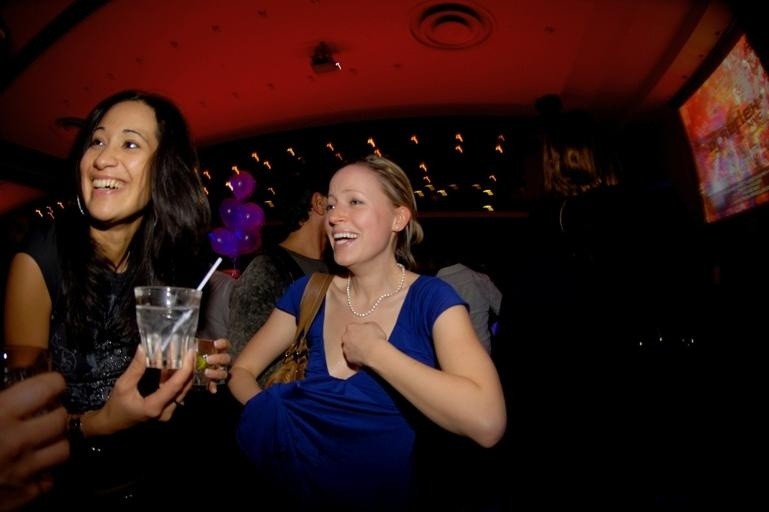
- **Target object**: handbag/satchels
[265,272,336,389]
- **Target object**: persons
[0,370,73,511]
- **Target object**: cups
[191,338,225,384]
[0,343,54,422]
[133,285,202,371]
